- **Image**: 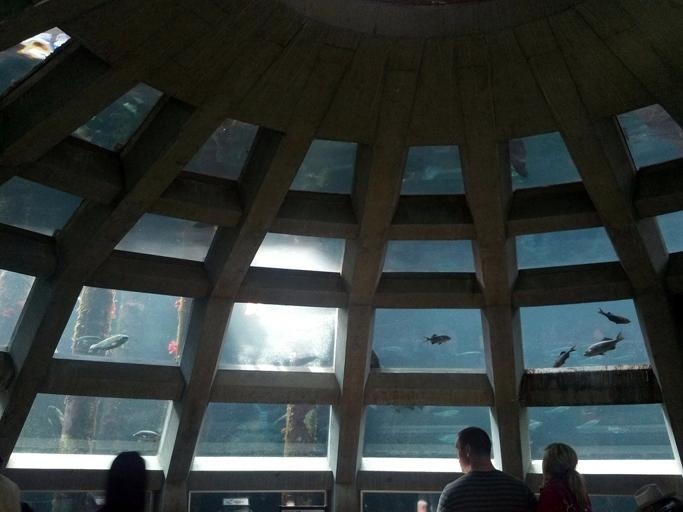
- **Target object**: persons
[0,351,22,512]
[436,426,536,512]
[633,482,683,512]
[537,443,593,512]
[97,451,149,512]
